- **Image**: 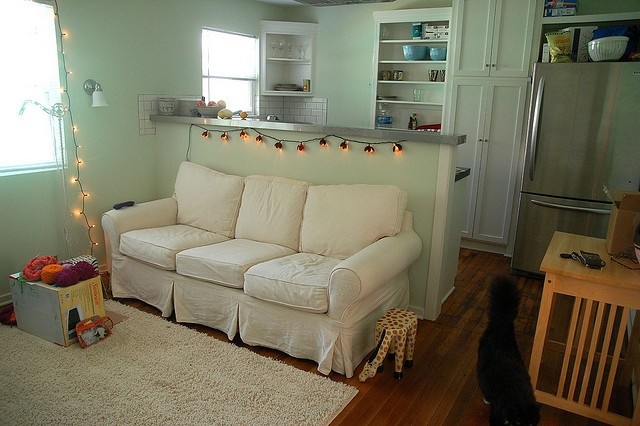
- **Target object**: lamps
[84,81,107,108]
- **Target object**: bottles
[376,106,393,127]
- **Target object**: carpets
[0,300,359,426]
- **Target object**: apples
[208,101,215,106]
[218,100,226,108]
[196,100,206,107]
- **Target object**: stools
[358,306,418,383]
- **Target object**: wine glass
[288,41,293,58]
[298,45,304,59]
[271,41,277,58]
[278,41,285,57]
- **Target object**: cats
[476,273,545,426]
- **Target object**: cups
[428,69,438,81]
[392,70,403,81]
[380,71,391,81]
[382,32,392,39]
[440,69,446,82]
[414,89,420,101]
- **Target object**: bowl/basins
[178,98,201,117]
[587,36,631,62]
[430,48,446,60]
[199,107,223,118]
[403,46,426,61]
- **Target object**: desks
[526,230,640,425]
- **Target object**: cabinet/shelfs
[452,0,537,77]
[259,19,314,98]
[448,79,529,253]
[539,1,639,64]
[369,7,456,132]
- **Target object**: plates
[275,83,300,91]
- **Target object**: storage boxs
[603,190,640,255]
[8,272,129,347]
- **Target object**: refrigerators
[508,64,640,279]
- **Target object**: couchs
[100,164,421,376]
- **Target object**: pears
[218,109,233,119]
